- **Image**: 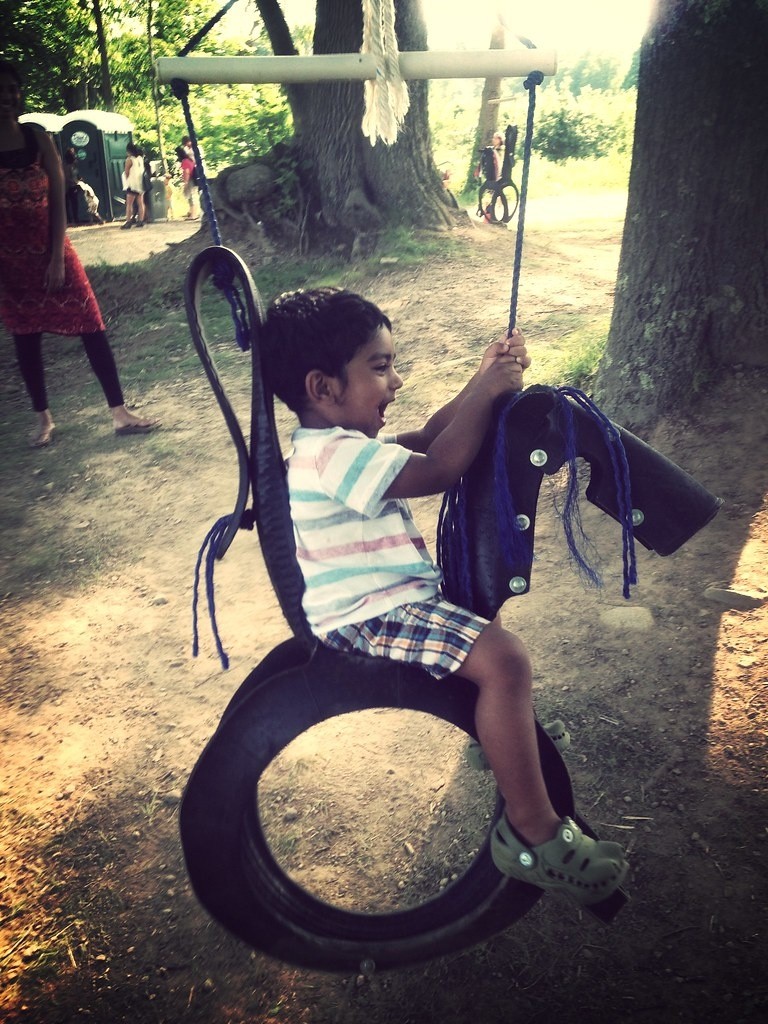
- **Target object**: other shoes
[185,216,199,220]
[182,212,192,217]
[136,220,144,228]
[120,220,132,230]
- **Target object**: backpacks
[143,170,151,191]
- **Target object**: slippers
[114,418,159,434]
[27,422,56,447]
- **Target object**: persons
[120,142,155,229]
[175,136,205,221]
[256,285,630,903]
[492,131,506,182]
[0,61,163,450]
[63,148,104,227]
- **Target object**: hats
[180,136,191,147]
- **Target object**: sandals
[467,718,570,772]
[491,815,628,904]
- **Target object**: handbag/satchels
[193,166,205,187]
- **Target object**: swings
[175,71,713,967]
